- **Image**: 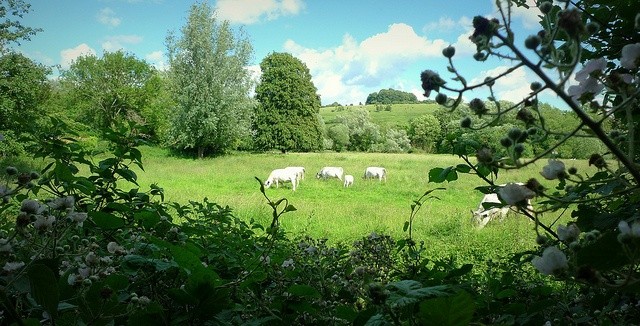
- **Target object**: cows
[362,167,388,183]
[470,182,536,227]
[342,174,355,189]
[315,166,344,182]
[262,166,305,193]
